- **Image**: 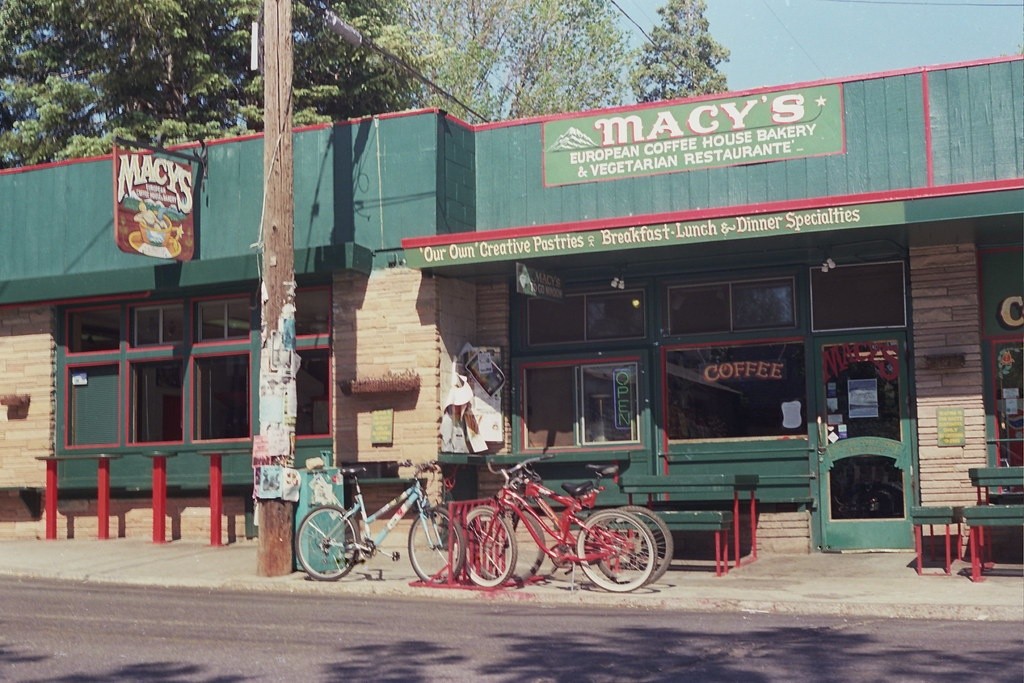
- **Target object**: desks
[965,467,1024,577]
[33,454,126,540]
[193,448,254,545]
[617,471,760,568]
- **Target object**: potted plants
[337,366,421,396]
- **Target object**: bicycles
[293,459,467,589]
[463,451,676,591]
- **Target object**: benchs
[909,502,966,576]
[960,506,1024,582]
[587,507,734,577]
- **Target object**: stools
[140,450,179,543]
